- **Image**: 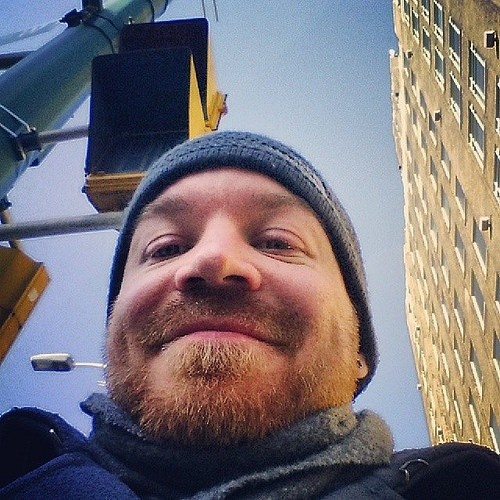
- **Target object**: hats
[106,129,379,401]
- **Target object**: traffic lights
[82,17,229,240]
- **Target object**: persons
[1,129,500,500]
